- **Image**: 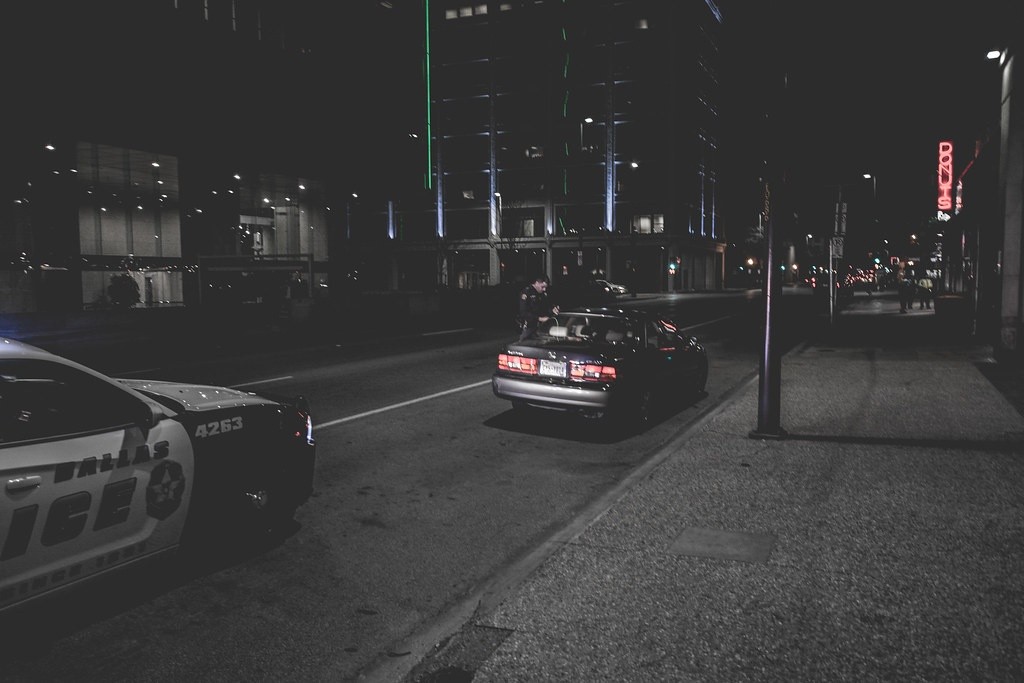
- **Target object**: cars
[596,279,628,294]
[491,308,708,426]
[0,339,318,616]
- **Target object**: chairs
[606,329,623,344]
[549,326,568,341]
[575,325,590,340]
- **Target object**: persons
[898,271,933,314]
[519,274,561,344]
